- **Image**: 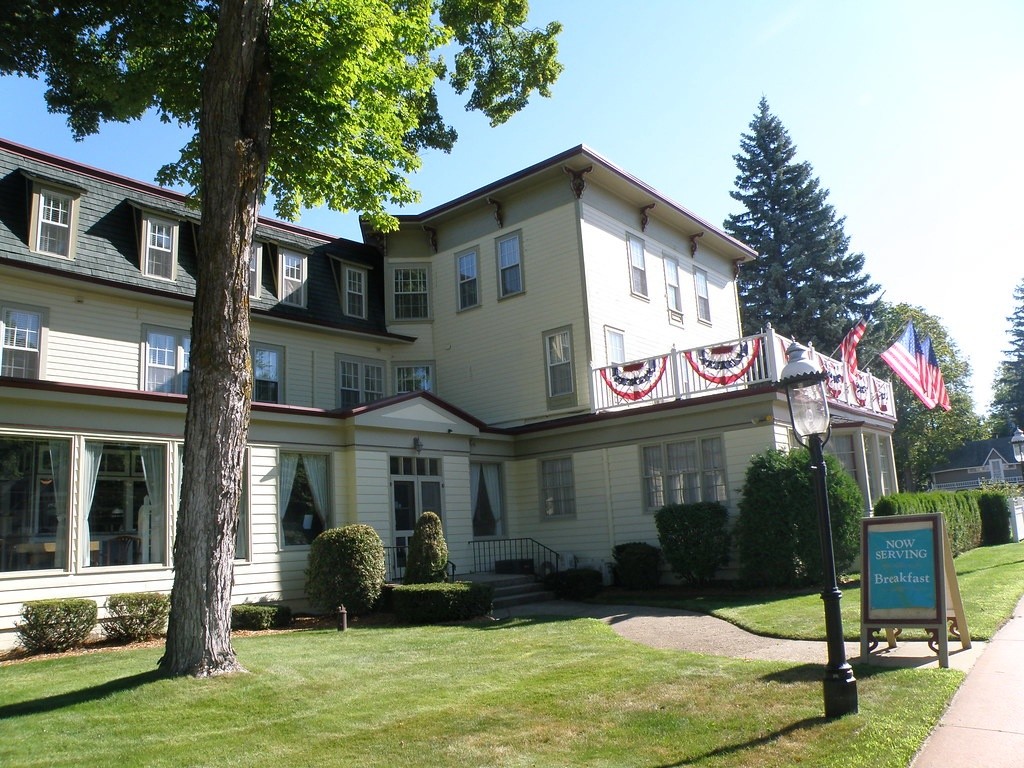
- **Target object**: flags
[879,317,951,411]
[839,310,870,407]
